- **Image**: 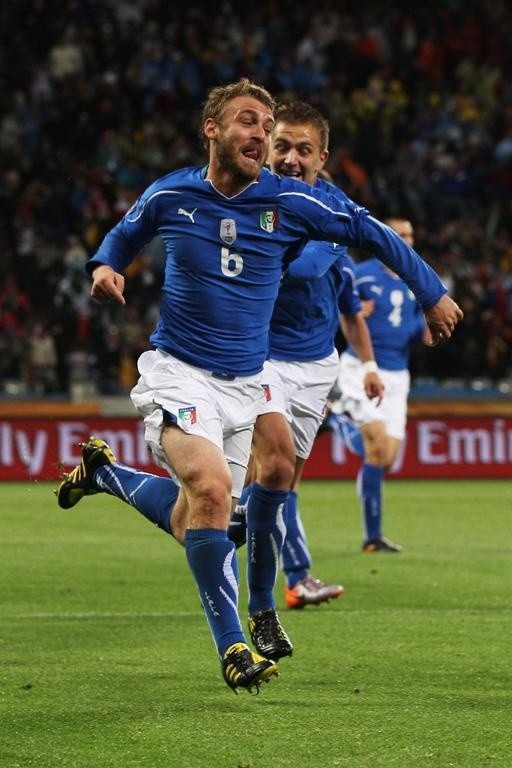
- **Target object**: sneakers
[57,438,117,509]
[363,539,402,552]
[219,608,294,690]
[284,574,343,608]
